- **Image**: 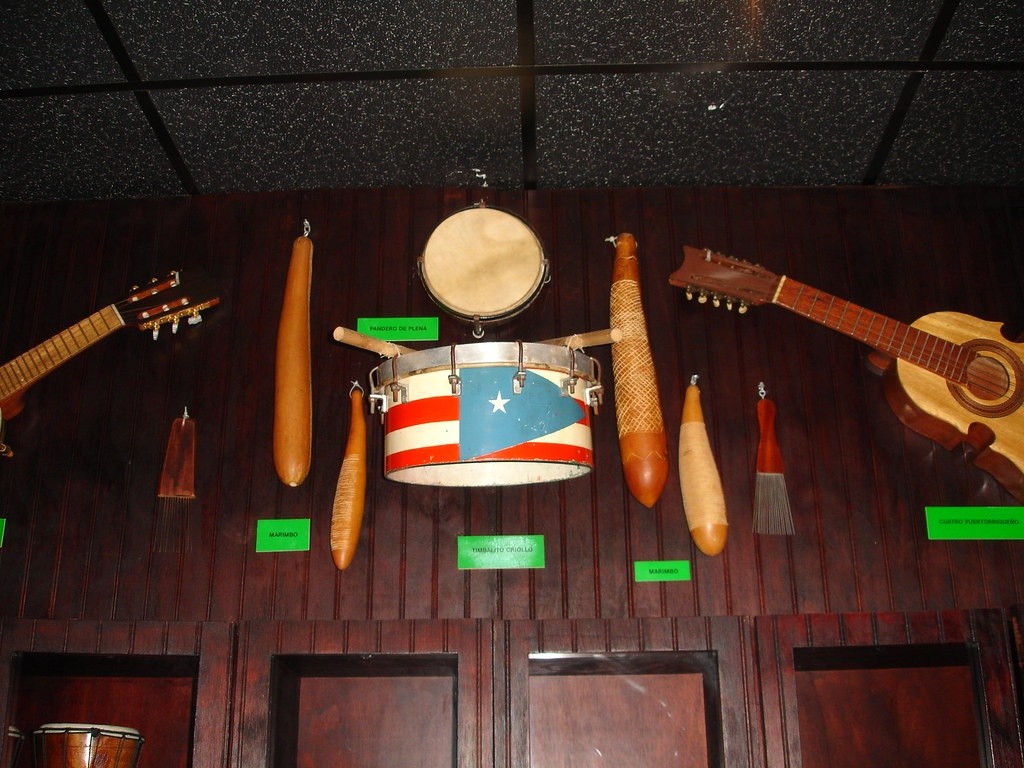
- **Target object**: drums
[373,341,604,491]
[39,722,146,768]
[414,209,553,325]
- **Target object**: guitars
[0,269,194,406]
[667,246,1024,506]
